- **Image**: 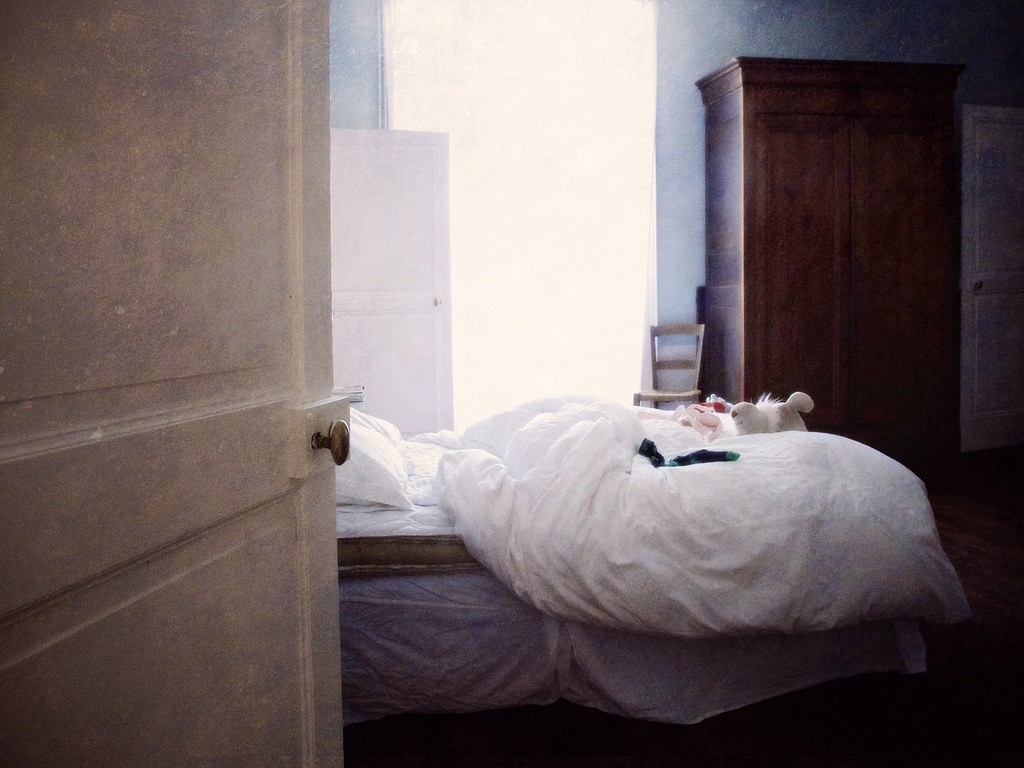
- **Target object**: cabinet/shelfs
[695,57,965,480]
[332,128,454,433]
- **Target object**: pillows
[329,407,417,511]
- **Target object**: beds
[341,406,970,730]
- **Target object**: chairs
[635,321,706,407]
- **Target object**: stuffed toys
[672,405,722,440]
[731,392,814,436]
[694,394,733,413]
